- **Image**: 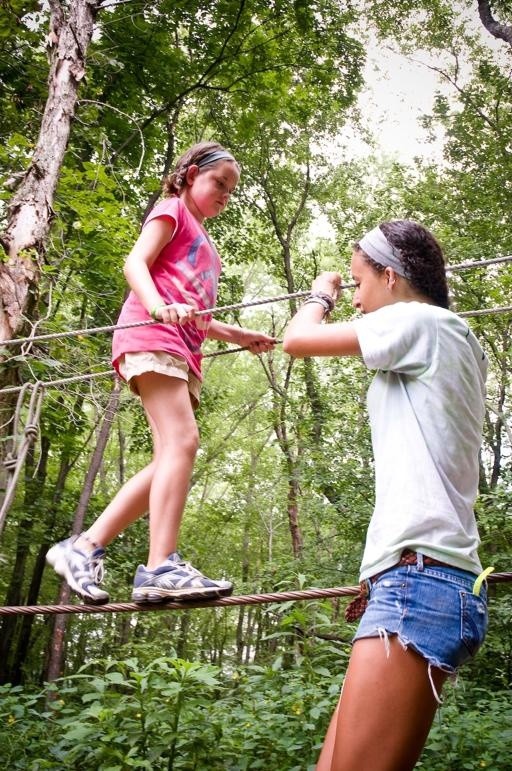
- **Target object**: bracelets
[151,304,166,321]
[302,293,336,317]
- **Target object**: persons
[43,142,277,606]
[283,221,490,771]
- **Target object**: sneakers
[132,552,233,604]
[45,534,110,604]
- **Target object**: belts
[344,550,448,622]
